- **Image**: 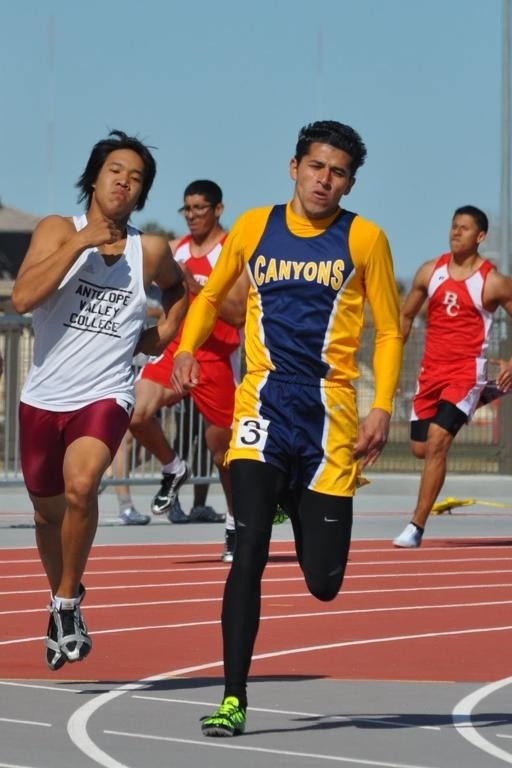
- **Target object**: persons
[13,130,189,669]
[170,120,407,735]
[392,205,512,548]
[166,398,227,523]
[96,279,162,523]
[130,180,247,514]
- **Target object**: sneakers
[392,523,422,548]
[117,505,150,525]
[189,505,226,523]
[221,527,238,563]
[43,583,92,671]
[151,460,191,523]
[200,696,246,737]
